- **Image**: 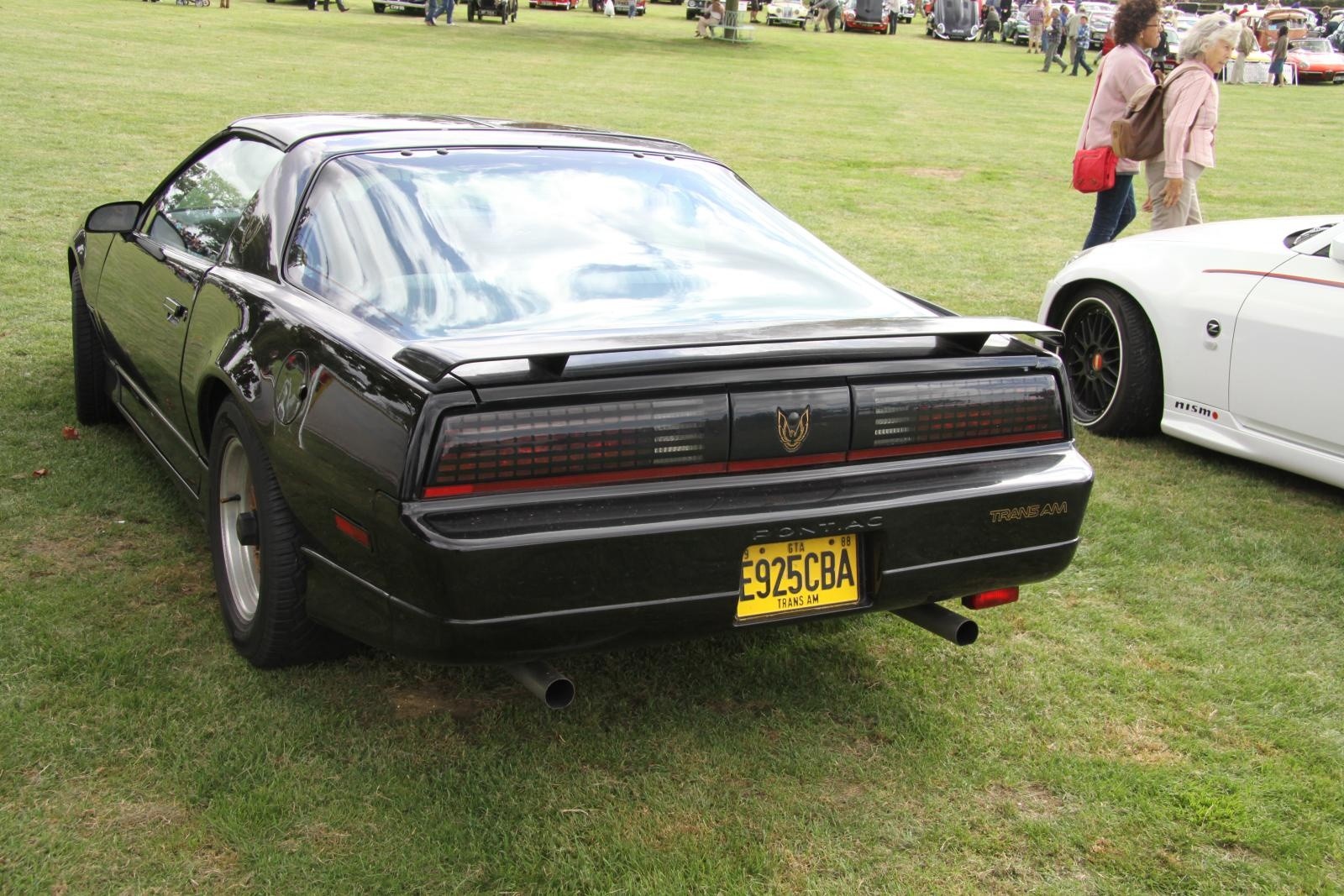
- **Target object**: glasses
[1205,20,1228,38]
[1146,20,1161,28]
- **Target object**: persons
[1072,0,1166,249]
[628,0,637,19]
[1315,5,1333,26]
[1224,2,1250,22]
[1093,26,1117,65]
[977,4,1011,43]
[423,0,456,26]
[603,0,616,17]
[1152,24,1167,73]
[1023,0,1094,76]
[1224,18,1254,85]
[1264,0,1283,10]
[1267,26,1289,87]
[1139,10,1242,230]
[811,0,840,33]
[750,0,761,23]
[323,0,350,12]
[1291,0,1302,9]
[694,0,726,38]
[887,0,900,34]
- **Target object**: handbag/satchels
[1072,147,1117,193]
[604,0,614,15]
[1042,31,1049,52]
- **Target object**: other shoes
[1087,70,1093,76]
[1062,65,1068,72]
[1069,72,1077,76]
[1035,50,1038,54]
[1027,49,1031,53]
[1038,69,1048,72]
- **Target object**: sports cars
[1035,207,1343,487]
[64,110,1094,715]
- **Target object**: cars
[921,0,1344,85]
[467,0,518,24]
[592,0,646,16]
[841,0,916,35]
[747,0,763,11]
[766,0,809,28]
[686,0,726,20]
[529,0,579,12]
[372,0,430,18]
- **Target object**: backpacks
[1112,66,1211,161]
